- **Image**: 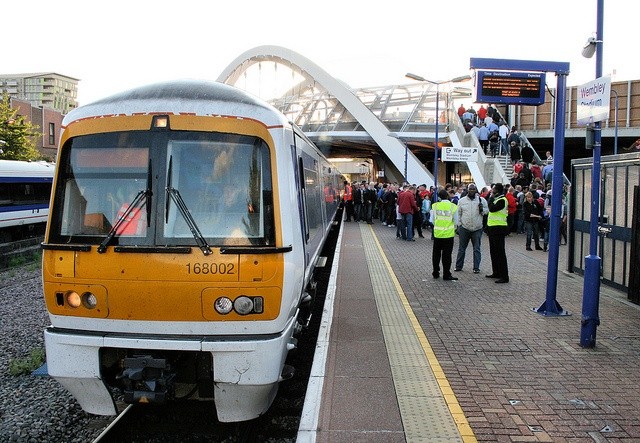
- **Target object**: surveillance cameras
[581,36,596,59]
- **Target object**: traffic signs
[441,146,478,161]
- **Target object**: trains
[0,159,56,231]
[41,79,346,422]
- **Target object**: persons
[342,181,396,228]
[398,182,421,191]
[429,190,459,280]
[421,194,431,230]
[420,184,431,199]
[396,187,420,242]
[430,183,468,205]
[209,184,250,237]
[483,183,510,283]
[107,183,147,235]
[506,151,569,252]
[458,103,532,151]
[453,183,489,273]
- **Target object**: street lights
[387,132,407,183]
[405,72,472,201]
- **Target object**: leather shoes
[433,274,440,279]
[443,275,459,280]
[454,267,462,271]
[495,277,509,284]
[344,219,351,222]
[473,268,480,274]
[485,274,496,278]
[367,221,374,224]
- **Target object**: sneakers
[526,246,532,251]
[382,222,387,226]
[420,235,424,238]
[396,237,400,239]
[407,239,415,242]
[402,237,407,240]
[535,245,543,250]
[392,224,395,228]
[388,225,391,227]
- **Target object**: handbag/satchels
[483,214,487,233]
